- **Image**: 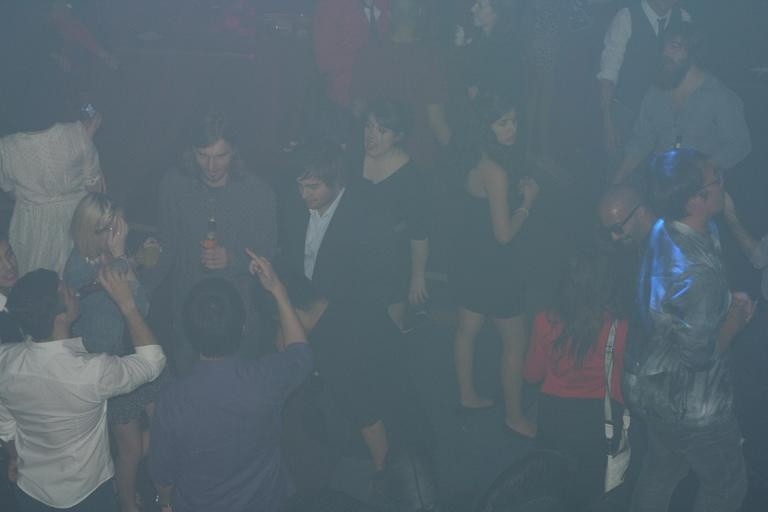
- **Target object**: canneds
[80,103,95,121]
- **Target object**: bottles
[73,259,128,300]
[202,218,219,251]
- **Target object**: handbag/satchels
[605,438,633,493]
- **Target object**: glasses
[703,173,723,190]
[604,202,641,234]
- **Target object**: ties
[657,15,666,41]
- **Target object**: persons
[0,0,767,512]
